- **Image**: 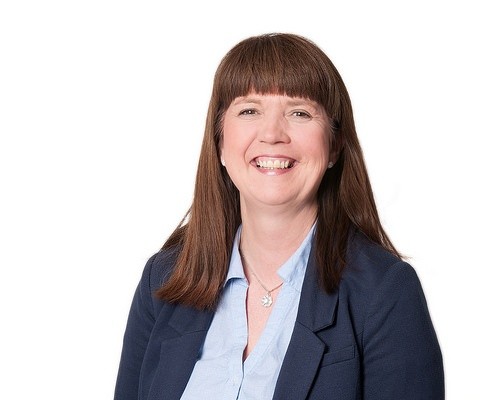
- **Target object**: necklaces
[238,247,284,309]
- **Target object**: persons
[113,33,447,400]
